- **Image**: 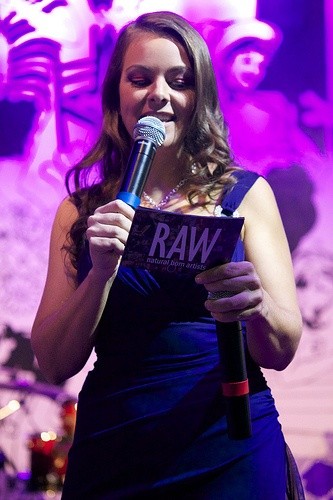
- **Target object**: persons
[27,10,307,500]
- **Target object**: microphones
[207,289,254,442]
[114,115,166,214]
